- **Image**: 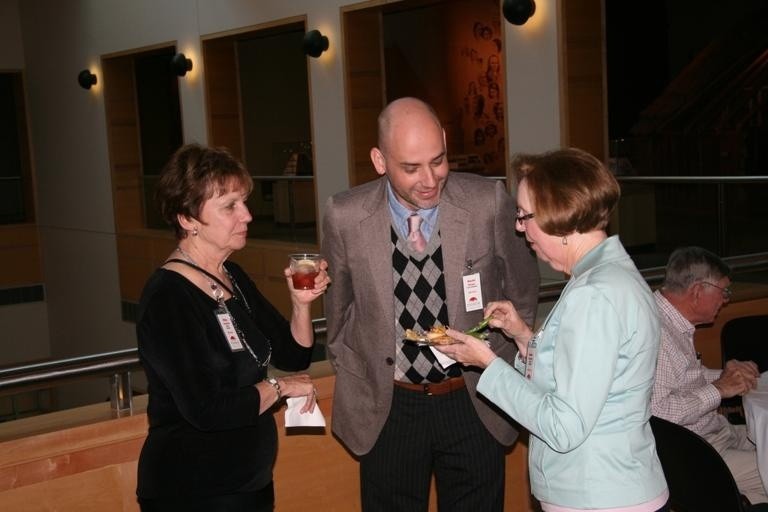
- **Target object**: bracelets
[260,377,281,403]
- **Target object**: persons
[133,142,333,510]
[430,148,677,511]
[639,241,768,509]
[319,96,539,511]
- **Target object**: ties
[406,214,427,256]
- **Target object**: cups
[288,252,326,291]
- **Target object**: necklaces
[175,245,274,370]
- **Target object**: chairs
[721,314,768,422]
[650,414,743,510]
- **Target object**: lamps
[303,30,329,57]
[78,70,97,89]
[502,0,536,25]
[172,54,192,76]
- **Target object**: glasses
[514,206,536,225]
[700,281,732,299]
